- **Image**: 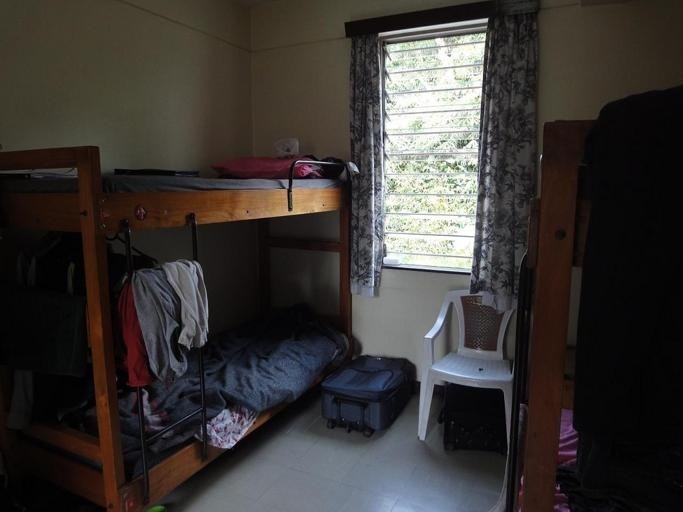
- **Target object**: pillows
[209,157,324,181]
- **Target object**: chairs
[416,289,517,455]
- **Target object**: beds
[1,143,353,510]
[512,118,683,511]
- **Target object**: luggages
[321,355,417,437]
